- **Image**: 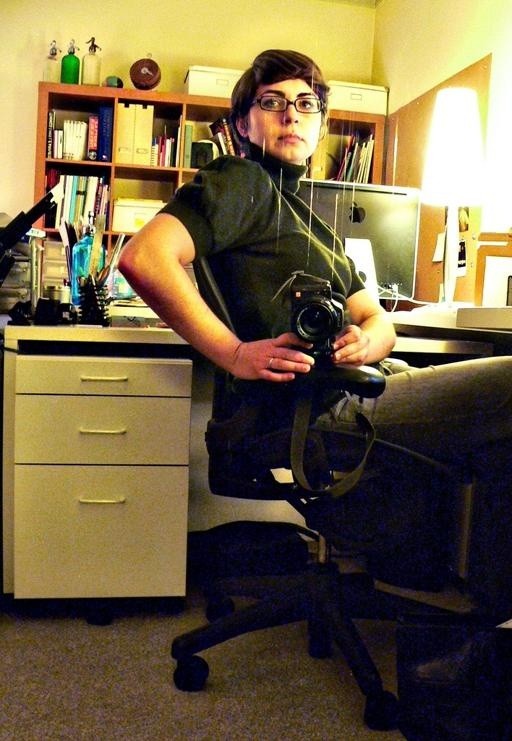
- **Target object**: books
[44,167,109,231]
[46,107,112,162]
[184,116,245,169]
[118,102,183,168]
[299,158,310,178]
[454,306,512,333]
[336,134,375,184]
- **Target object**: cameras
[292,272,345,360]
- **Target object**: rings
[267,356,274,368]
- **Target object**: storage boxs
[181,65,245,97]
[112,197,167,233]
[327,80,390,118]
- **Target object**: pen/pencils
[64,215,126,304]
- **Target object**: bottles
[70,210,106,306]
[43,37,102,87]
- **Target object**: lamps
[412,86,486,314]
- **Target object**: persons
[117,46,512,476]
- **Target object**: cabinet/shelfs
[34,80,392,323]
[1,346,195,628]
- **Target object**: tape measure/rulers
[90,215,106,277]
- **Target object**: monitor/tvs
[298,178,421,305]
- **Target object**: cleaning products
[80,36,103,86]
[60,39,81,85]
[43,39,63,84]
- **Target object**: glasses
[248,95,325,113]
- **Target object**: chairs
[170,255,512,732]
